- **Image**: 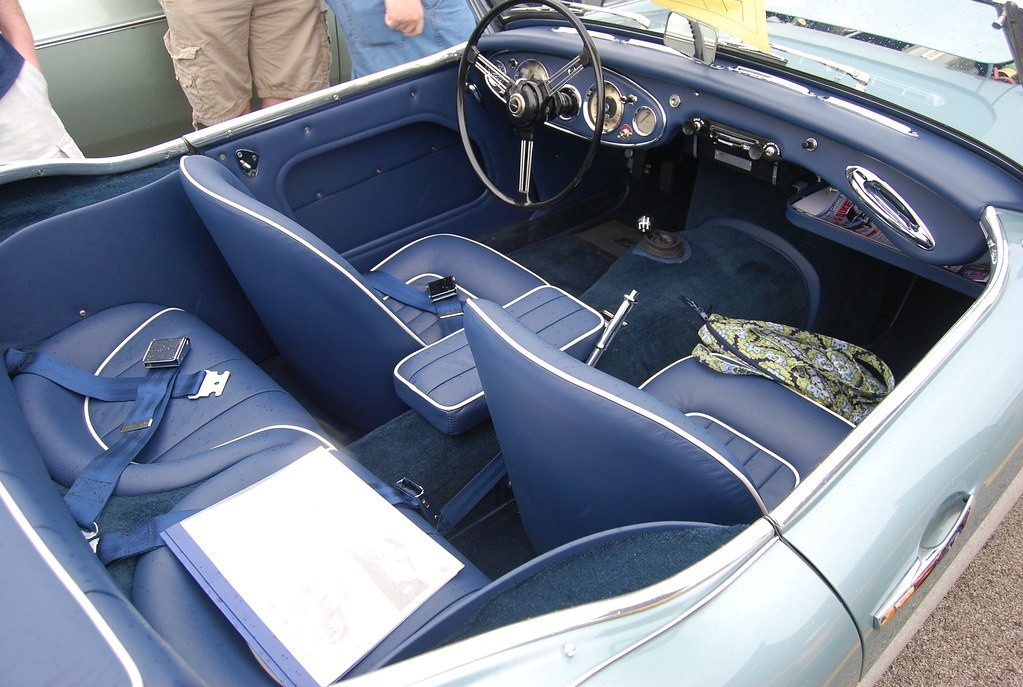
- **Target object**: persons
[0,0,85,162]
[157,0,334,132]
[326,0,477,81]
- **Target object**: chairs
[463,296,857,556]
[179,152,551,432]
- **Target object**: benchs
[0,299,489,687]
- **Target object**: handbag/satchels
[684,297,895,425]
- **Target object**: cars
[2,0,1023,687]
